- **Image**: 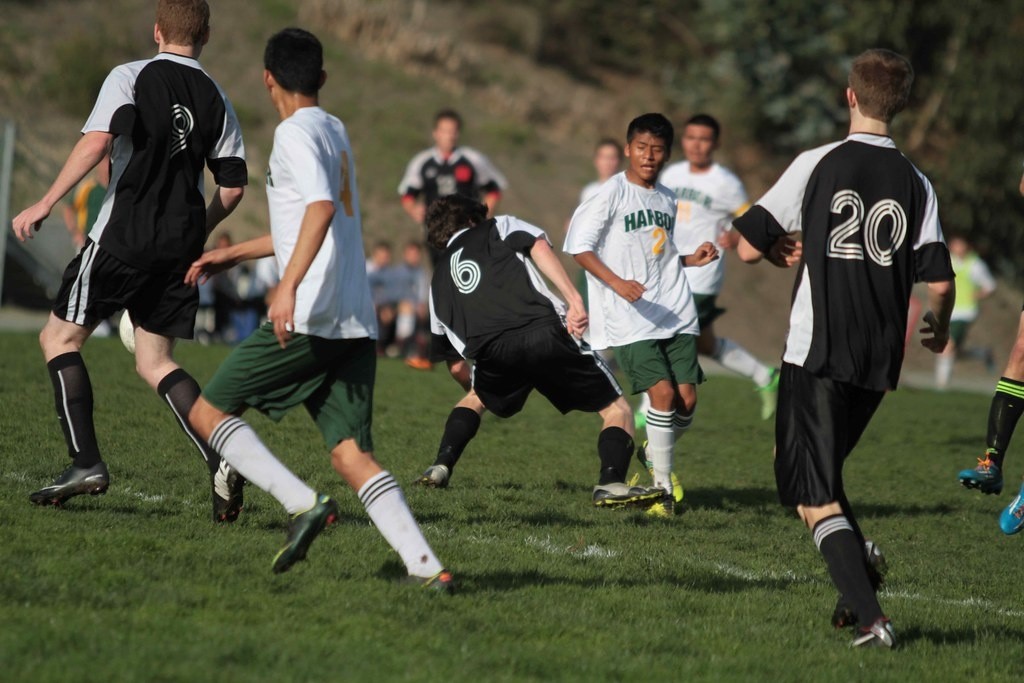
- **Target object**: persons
[632,114,781,431]
[931,234,991,387]
[195,232,278,346]
[563,112,719,517]
[731,48,957,649]
[12,0,249,522]
[183,28,455,592]
[956,303,1024,537]
[564,139,622,229]
[62,156,109,336]
[397,109,506,216]
[416,194,669,512]
[367,240,433,368]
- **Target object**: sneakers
[592,473,667,507]
[848,617,903,652]
[754,367,781,421]
[404,569,454,597]
[637,440,684,502]
[999,483,1024,535]
[958,448,1003,495]
[30,461,109,507]
[646,490,692,516]
[831,541,888,629]
[211,457,246,524]
[271,492,339,574]
[410,465,449,489]
